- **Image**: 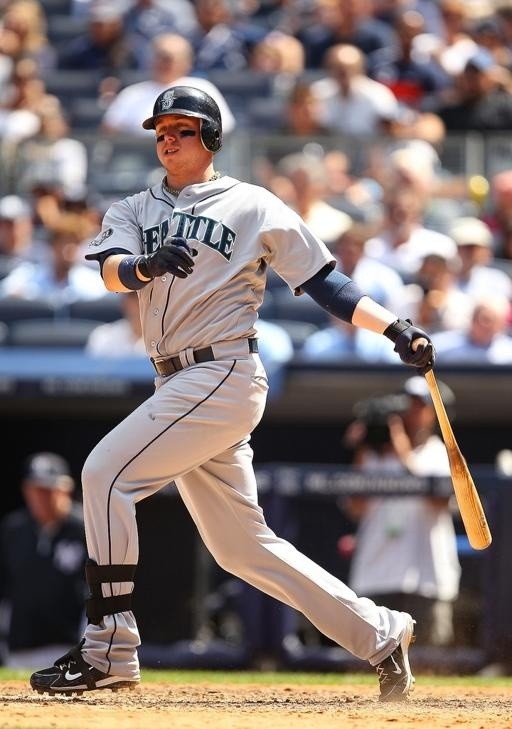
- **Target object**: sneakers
[375,612,416,702]
[30,655,140,696]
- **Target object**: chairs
[0,0,512,369]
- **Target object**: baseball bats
[412,338,492,551]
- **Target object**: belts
[154,338,259,378]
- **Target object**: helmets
[142,86,222,153]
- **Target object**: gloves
[138,236,198,279]
[383,318,438,376]
